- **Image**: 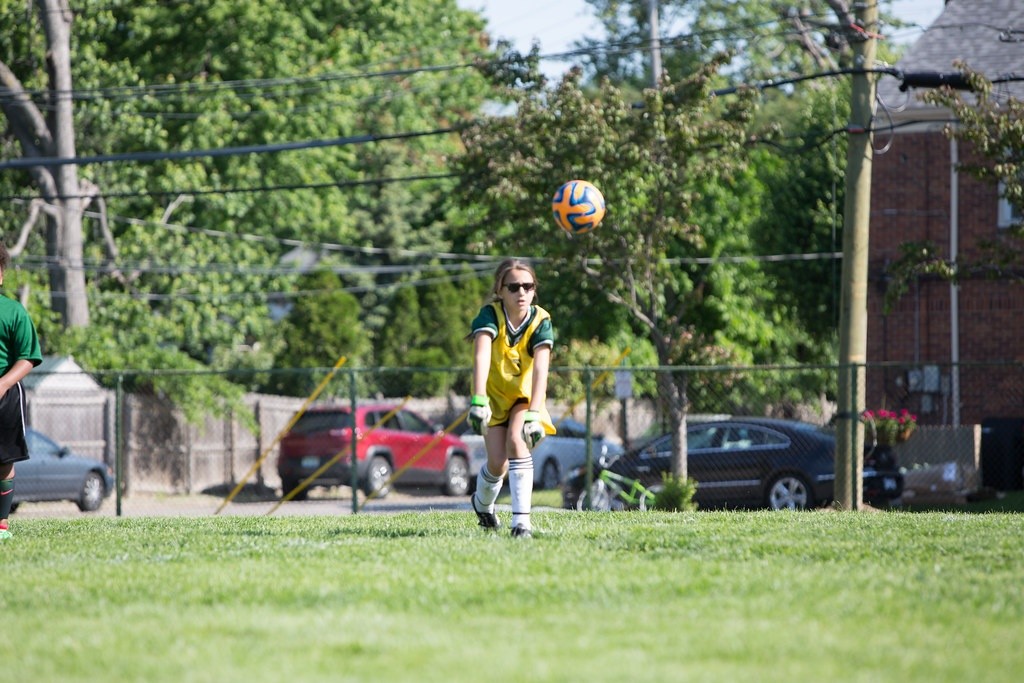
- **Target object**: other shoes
[0,528,11,539]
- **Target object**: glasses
[502,281,536,293]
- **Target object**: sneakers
[511,522,531,540]
[470,492,500,528]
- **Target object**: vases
[865,425,915,470]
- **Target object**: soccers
[551,178,606,235]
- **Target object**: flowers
[863,409,917,442]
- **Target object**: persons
[467,260,557,537]
[0,244,42,537]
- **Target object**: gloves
[467,394,492,435]
[518,411,545,450]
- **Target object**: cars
[0,428,114,512]
[460,417,626,491]
[562,419,877,510]
[278,405,471,498]
